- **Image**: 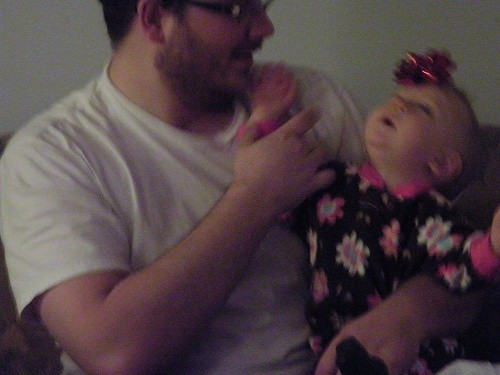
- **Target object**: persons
[0,1,471,375]
[234,48,500,375]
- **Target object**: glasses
[185,0,272,17]
